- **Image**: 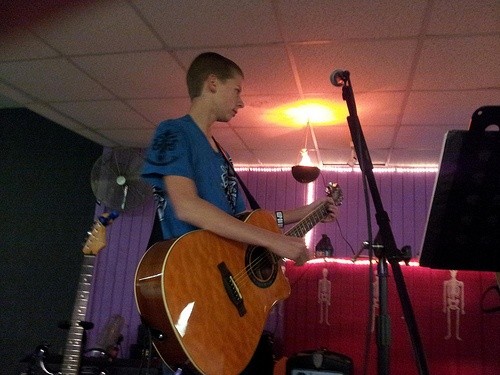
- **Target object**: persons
[139,52,339,267]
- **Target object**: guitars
[24,219,108,375]
[133,182,344,375]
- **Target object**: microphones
[331,70,350,87]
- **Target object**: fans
[90,145,154,211]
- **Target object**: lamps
[292,120,321,184]
